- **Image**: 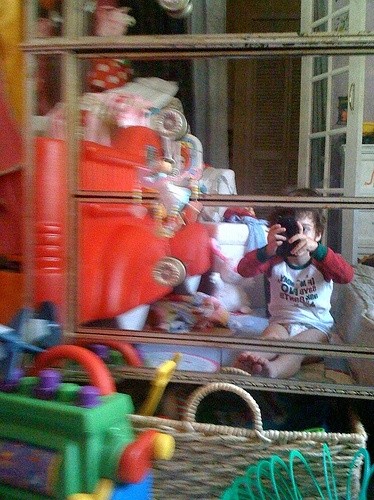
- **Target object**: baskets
[123,366,369,500]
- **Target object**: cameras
[276,216,300,256]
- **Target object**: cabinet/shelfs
[336,144,374,265]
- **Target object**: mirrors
[58,201,374,403]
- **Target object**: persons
[237,188,356,379]
